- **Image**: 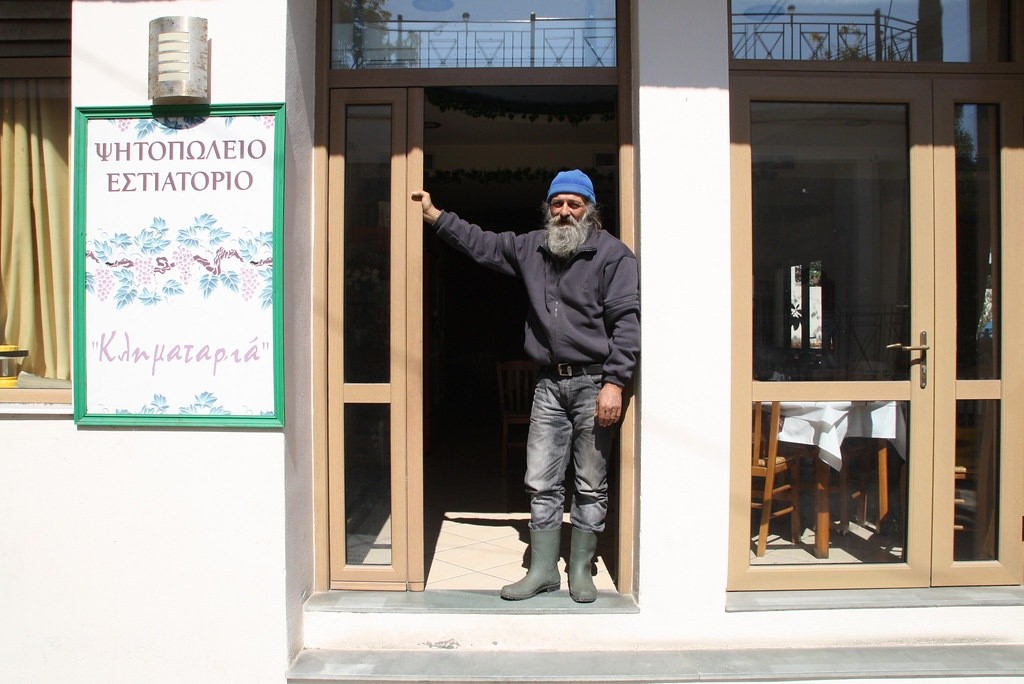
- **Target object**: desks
[754,401,907,559]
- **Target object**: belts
[547,362,604,378]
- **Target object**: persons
[410,167,641,606]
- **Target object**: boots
[500,527,561,600]
[568,526,598,602]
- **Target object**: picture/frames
[74,103,285,429]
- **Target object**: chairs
[752,405,799,556]
[496,360,538,472]
[905,329,993,562]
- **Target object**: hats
[546,169,596,207]
